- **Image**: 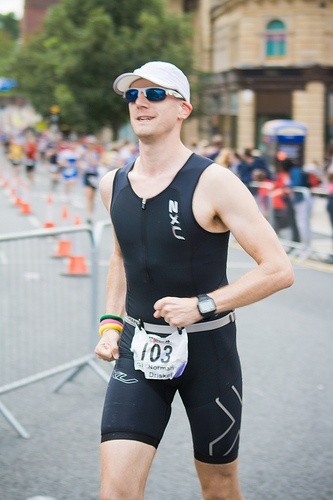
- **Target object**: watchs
[196,293,218,321]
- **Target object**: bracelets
[97,314,123,336]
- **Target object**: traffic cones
[53,207,72,259]
[41,197,56,229]
[0,168,24,210]
[64,216,90,275]
[17,183,32,215]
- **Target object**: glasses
[122,87,184,102]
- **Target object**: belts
[122,312,235,334]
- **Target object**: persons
[0,122,333,264]
[95,61,294,500]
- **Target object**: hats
[113,61,190,104]
